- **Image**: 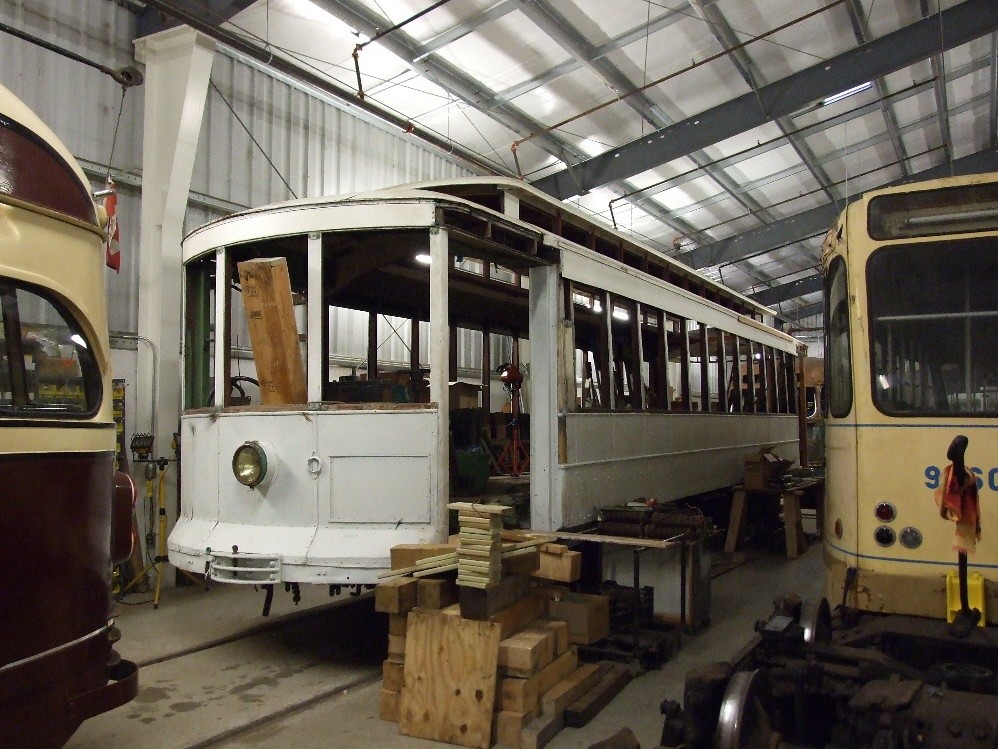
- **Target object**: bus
[1,84,998,746]
